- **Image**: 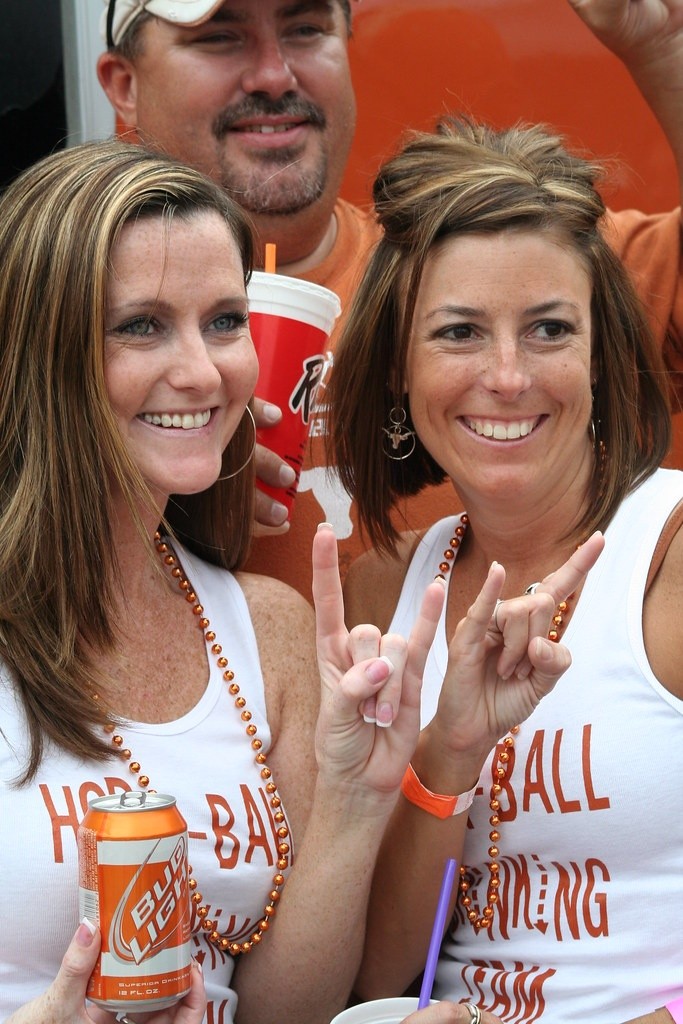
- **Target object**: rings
[462,1002,482,1024]
[120,1016,137,1024]
[492,598,505,632]
[525,582,540,595]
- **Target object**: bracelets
[402,763,480,818]
[666,997,683,1024]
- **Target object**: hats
[98,0,221,46]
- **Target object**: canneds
[77,791,195,1013]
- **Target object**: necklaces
[76,533,287,954]
[436,439,612,929]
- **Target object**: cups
[247,268,343,523]
[330,995,440,1024]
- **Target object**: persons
[314,111,683,1024]
[94,0,683,599]
[0,141,446,1024]
